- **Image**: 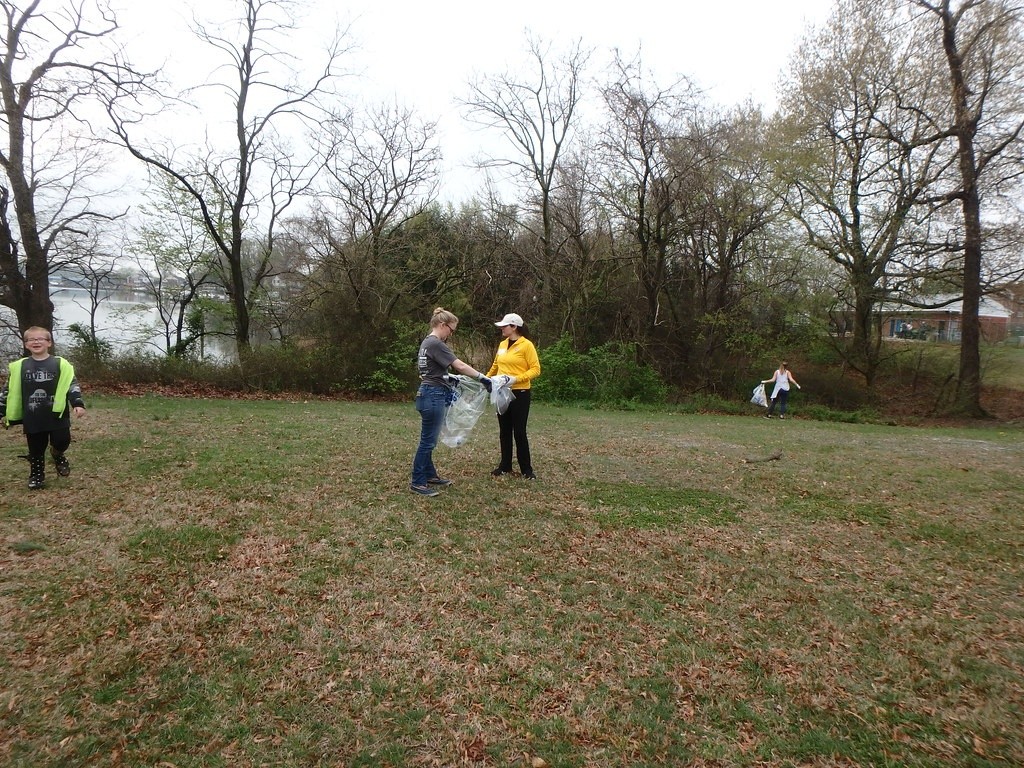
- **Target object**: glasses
[26,336,49,343]
[440,322,455,336]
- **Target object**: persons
[408,307,492,497]
[761,361,801,421]
[0,326,86,491]
[485,313,541,480]
[897,320,913,340]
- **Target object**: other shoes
[522,472,536,480]
[763,414,772,419]
[779,414,784,419]
[491,469,512,476]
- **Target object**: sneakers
[409,485,439,497]
[427,477,452,486]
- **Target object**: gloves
[443,374,461,387]
[761,381,766,384]
[797,384,801,389]
[474,372,492,393]
[501,375,515,387]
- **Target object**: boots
[28,455,45,489]
[50,447,70,476]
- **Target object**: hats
[494,313,524,327]
